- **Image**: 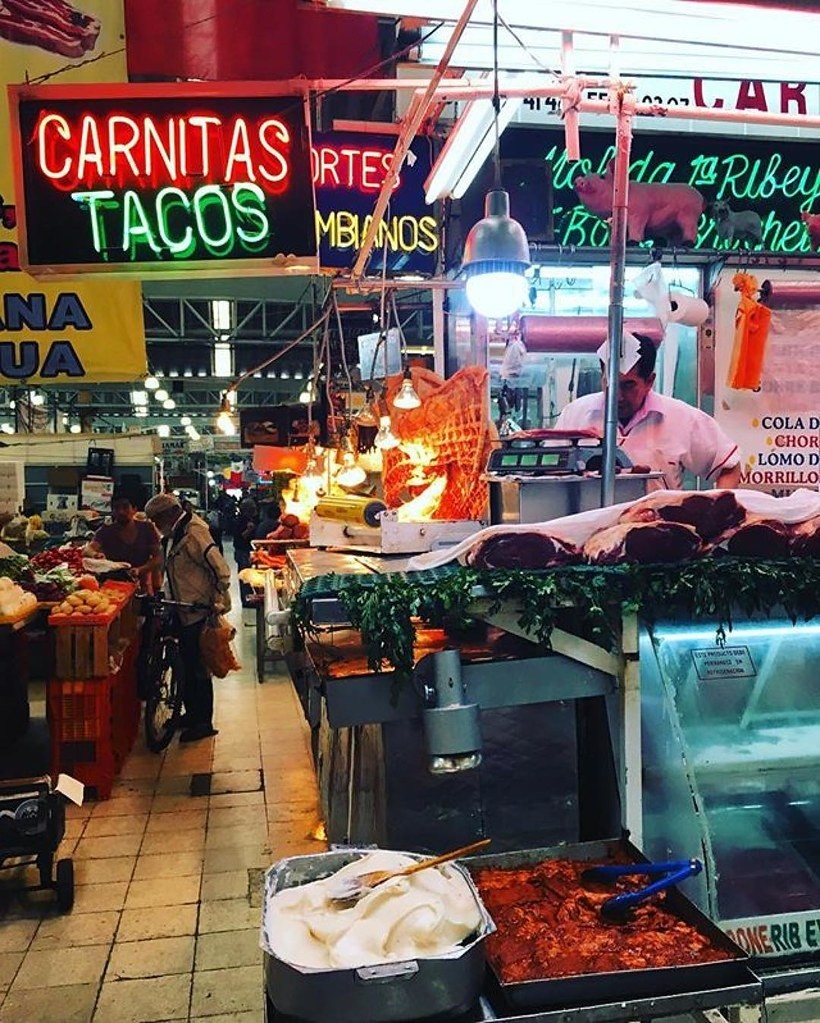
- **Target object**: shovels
[330,840,491,902]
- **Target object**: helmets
[239,498,257,509]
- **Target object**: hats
[145,493,179,520]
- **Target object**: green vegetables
[0,555,45,580]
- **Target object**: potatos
[50,589,110,614]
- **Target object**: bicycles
[135,590,215,750]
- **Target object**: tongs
[581,859,703,917]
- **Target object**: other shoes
[242,602,256,609]
[161,715,189,728]
[179,729,218,743]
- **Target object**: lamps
[423,70,526,205]
[285,290,421,518]
[460,0,531,317]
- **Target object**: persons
[88,492,232,742]
[543,332,742,494]
[183,489,282,541]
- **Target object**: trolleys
[0,782,99,911]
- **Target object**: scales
[485,435,634,472]
